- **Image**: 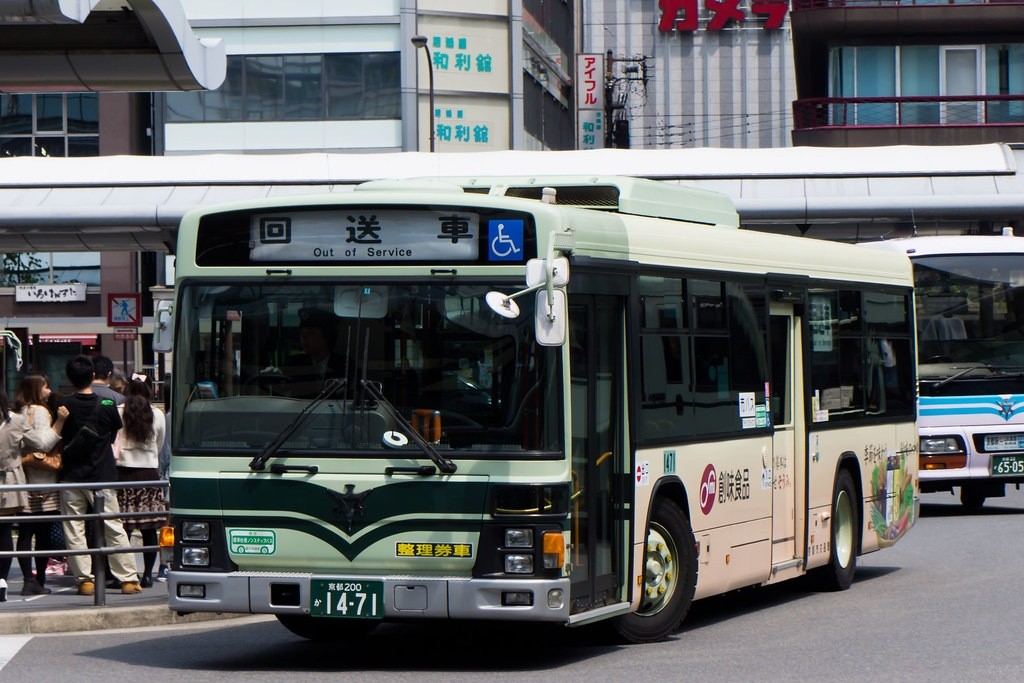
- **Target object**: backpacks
[62,413,113,485]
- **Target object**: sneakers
[121,583,142,594]
[78,581,95,596]
[22,577,51,595]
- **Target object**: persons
[0,356,171,601]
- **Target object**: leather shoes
[140,576,153,588]
[105,579,122,588]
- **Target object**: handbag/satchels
[22,445,64,469]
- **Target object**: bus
[853,235,1024,511]
[161,174,921,641]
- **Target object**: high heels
[0,578,8,602]
